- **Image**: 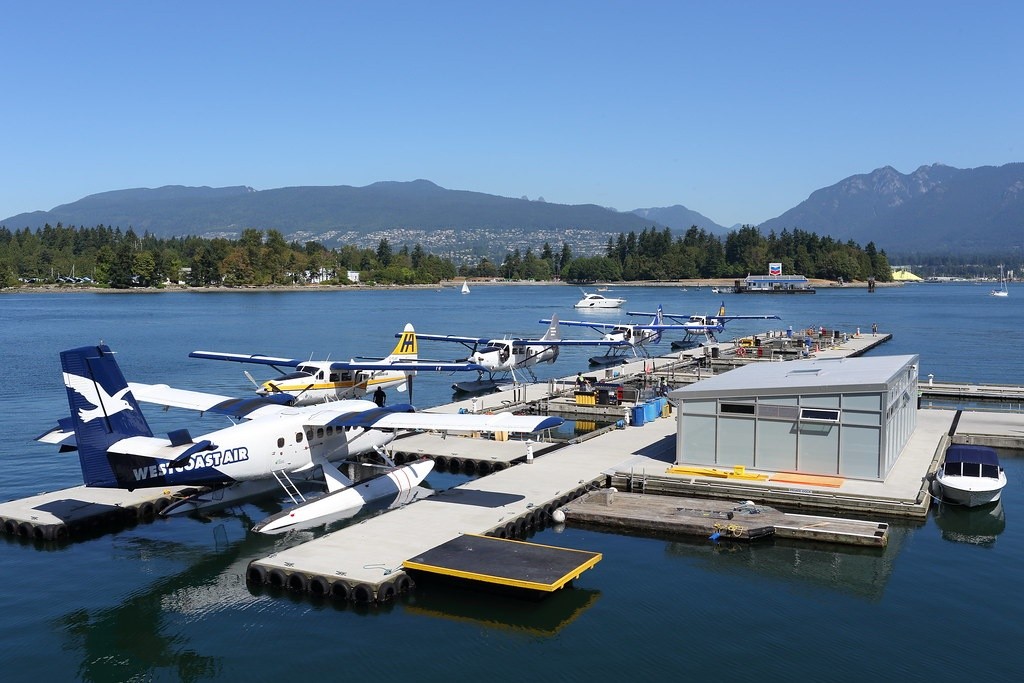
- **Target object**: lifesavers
[737,348,746,355]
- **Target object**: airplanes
[33,305,783,540]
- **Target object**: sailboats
[993,263,1009,297]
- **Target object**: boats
[713,271,817,295]
[936,446,1007,506]
[576,294,627,308]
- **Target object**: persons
[871,323,878,337]
[372,386,386,407]
[660,377,668,396]
[574,372,592,392]
